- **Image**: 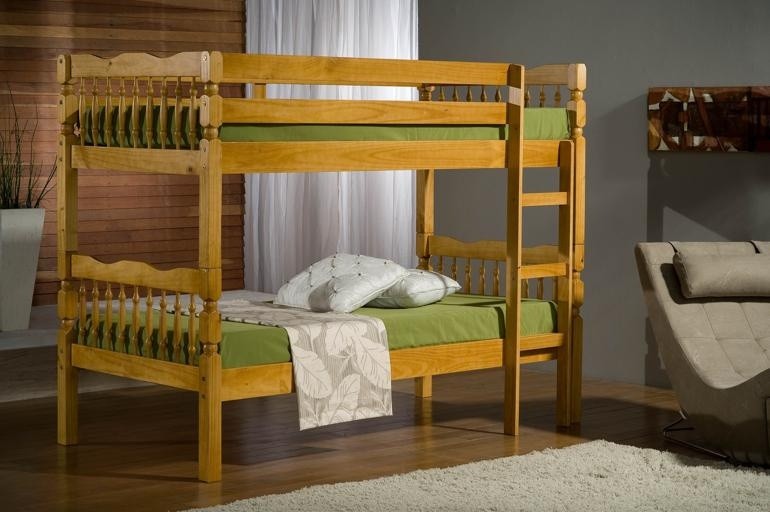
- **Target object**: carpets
[182,436,770,512]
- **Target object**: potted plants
[0,77,59,332]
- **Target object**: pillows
[272,250,463,316]
[673,250,769,299]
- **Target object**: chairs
[632,239,770,469]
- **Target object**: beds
[55,50,586,485]
